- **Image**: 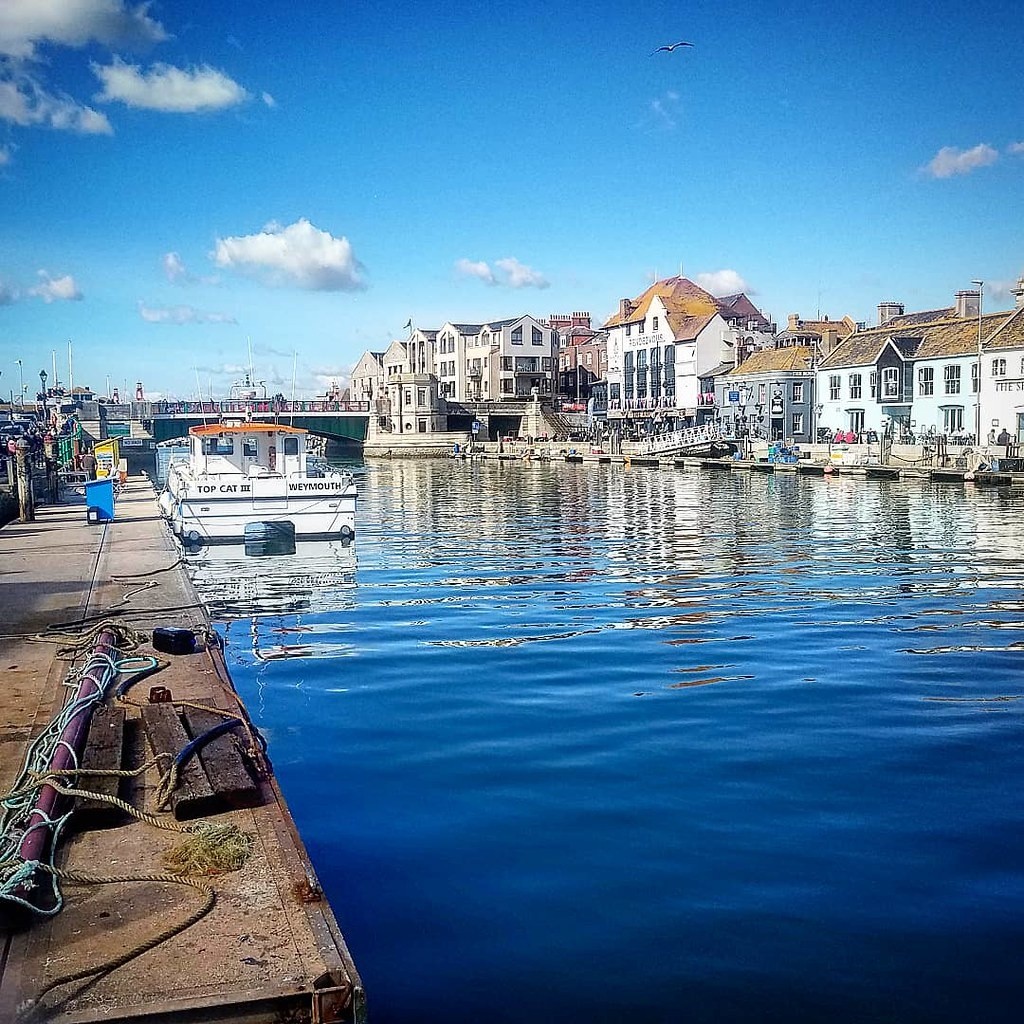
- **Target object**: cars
[0,408,39,454]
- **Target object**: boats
[165,502,356,605]
[564,454,583,463]
[166,339,359,501]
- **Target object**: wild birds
[648,42,695,57]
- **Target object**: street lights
[39,369,48,410]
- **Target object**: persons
[834,427,858,445]
[107,463,120,494]
[989,428,1011,446]
[80,450,98,482]
[35,426,44,467]
[5,435,16,456]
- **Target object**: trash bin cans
[85,478,113,524]
